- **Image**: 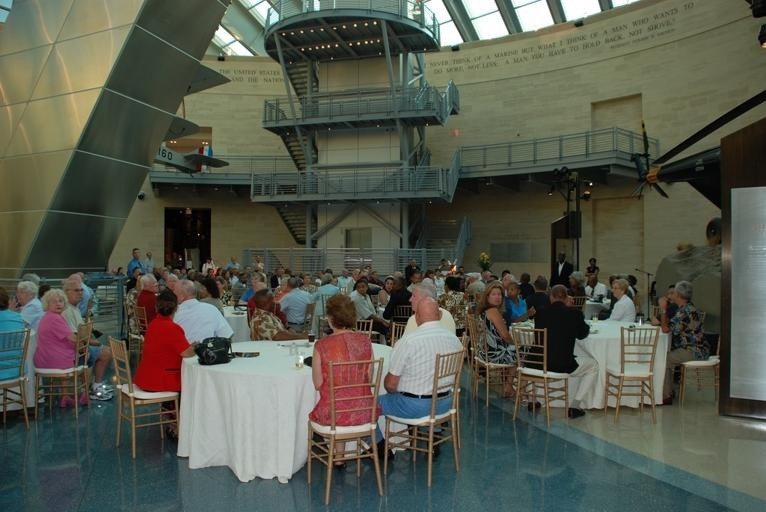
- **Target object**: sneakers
[527,401,542,412]
[59,398,76,408]
[375,439,395,464]
[567,407,586,419]
[662,389,677,405]
[89,381,114,402]
[426,429,443,461]
[79,391,91,405]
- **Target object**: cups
[307,329,316,344]
[371,289,391,310]
[637,316,644,326]
[587,293,604,303]
[593,313,599,322]
[294,352,304,368]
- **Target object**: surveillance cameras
[137,192,145,200]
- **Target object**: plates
[511,318,534,328]
[222,305,247,316]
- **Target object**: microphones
[635,268,641,272]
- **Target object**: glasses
[65,288,84,293]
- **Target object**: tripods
[643,272,653,322]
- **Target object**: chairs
[355,294,413,347]
[108,336,179,459]
[511,325,570,427]
[302,302,317,332]
[604,326,664,422]
[307,356,384,504]
[454,276,720,407]
[339,286,346,294]
[123,298,148,368]
[385,350,465,487]
[317,315,330,338]
[0,274,94,432]
[322,295,334,316]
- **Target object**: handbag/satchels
[193,336,233,366]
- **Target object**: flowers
[472,248,495,270]
